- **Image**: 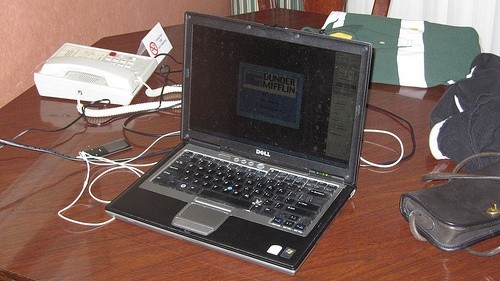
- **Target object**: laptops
[105,11,373,276]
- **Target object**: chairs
[258,0,391,18]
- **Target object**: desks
[0,8,500,281]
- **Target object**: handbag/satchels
[399,152,500,256]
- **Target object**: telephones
[33,42,183,117]
